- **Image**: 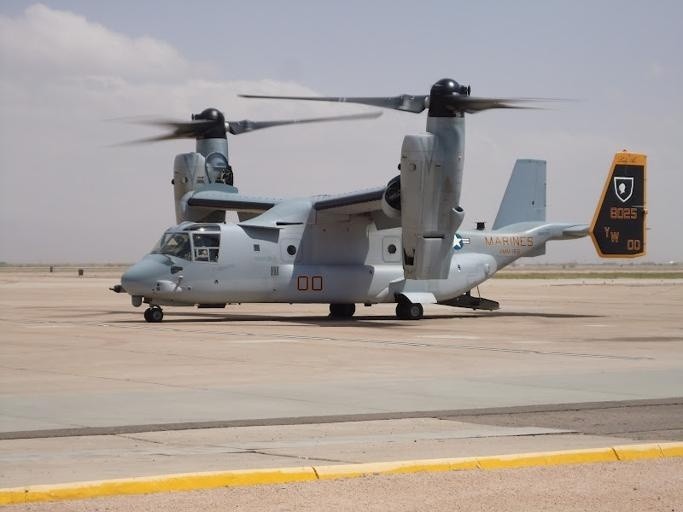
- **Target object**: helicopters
[104,78,648,322]
